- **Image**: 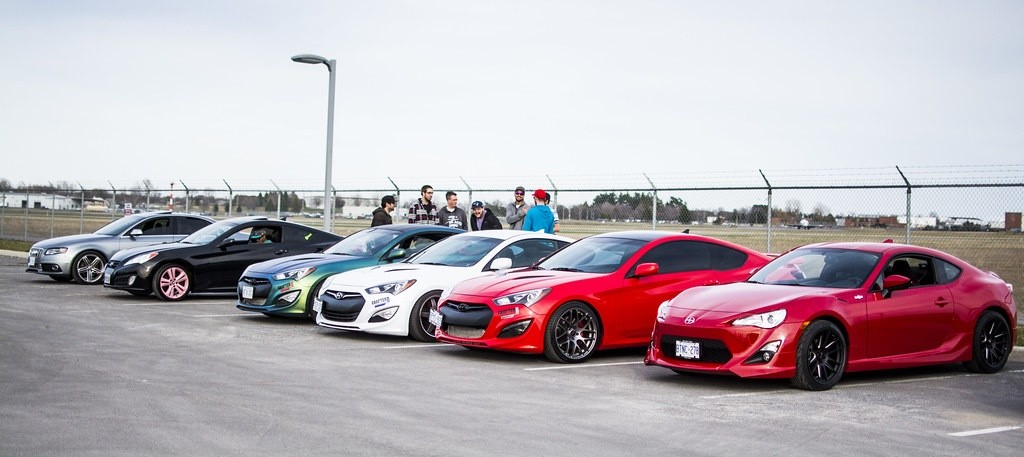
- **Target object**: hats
[516,187,525,193]
[532,190,546,199]
[471,201,483,209]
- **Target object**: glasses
[516,192,524,196]
[427,192,434,194]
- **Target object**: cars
[316,229,623,343]
[644,239,1017,391]
[102,216,345,301]
[435,229,806,364]
[236,224,524,322]
[25,212,216,285]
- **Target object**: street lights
[291,54,336,232]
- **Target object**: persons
[371,196,397,227]
[506,187,529,230]
[255,230,272,243]
[470,201,502,231]
[522,189,554,234]
[408,185,439,225]
[545,193,560,235]
[438,191,468,230]
